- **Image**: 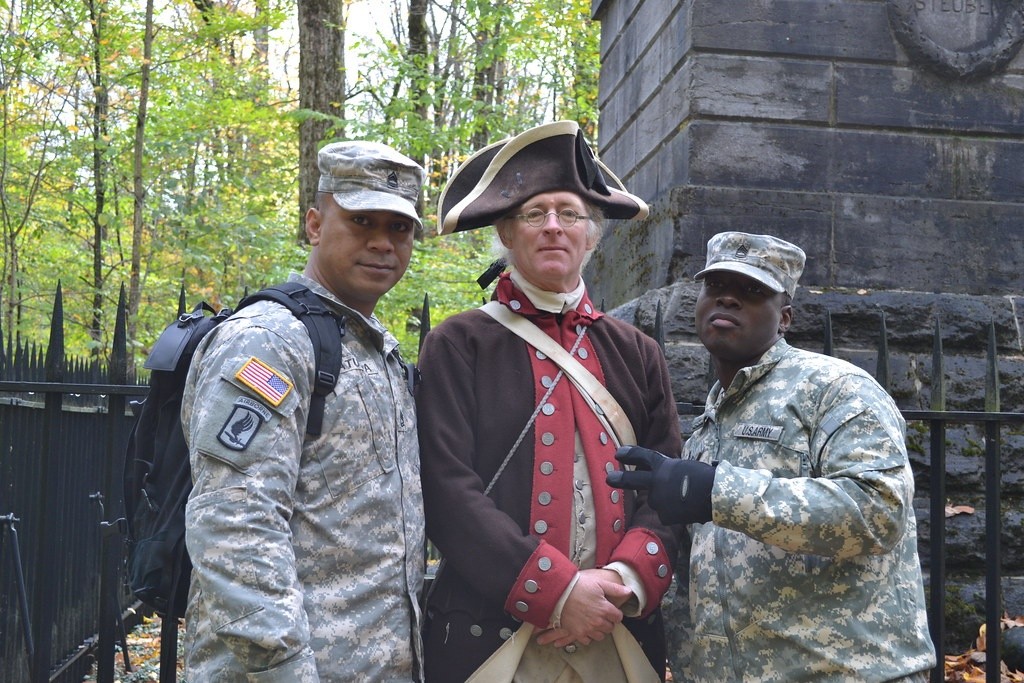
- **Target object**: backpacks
[128,283,342,683]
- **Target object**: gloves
[605,444,719,525]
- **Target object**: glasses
[505,207,592,229]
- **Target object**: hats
[438,120,649,236]
[316,139,428,239]
[693,233,805,299]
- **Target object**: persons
[415,120,684,683]
[179,141,432,683]
[607,232,938,683]
